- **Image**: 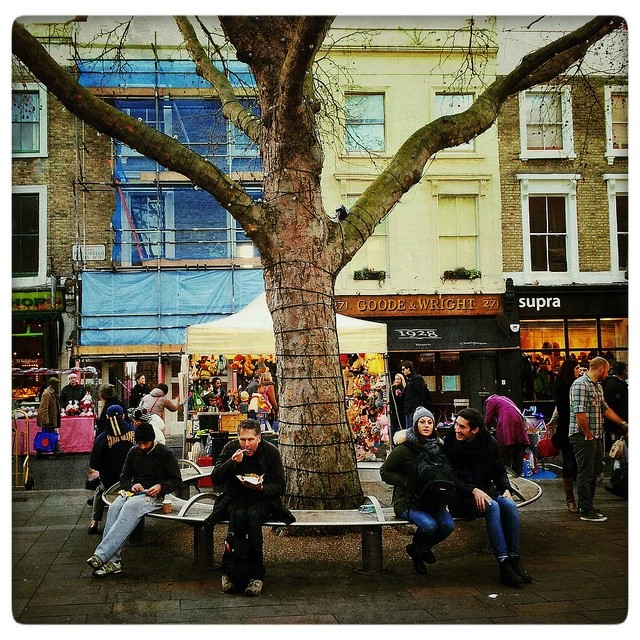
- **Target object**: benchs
[102,466,542,570]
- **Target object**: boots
[509,554,532,583]
[497,556,524,585]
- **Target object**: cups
[161,500,173,513]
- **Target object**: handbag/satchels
[33,431,58,452]
[85,478,100,490]
[537,438,560,458]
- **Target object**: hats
[49,377,61,384]
[412,406,435,433]
[131,407,151,421]
[107,405,123,418]
[134,422,155,441]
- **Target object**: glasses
[402,368,405,371]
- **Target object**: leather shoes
[36,455,48,459]
[55,452,67,458]
[88,524,98,534]
[566,499,578,512]
[414,542,436,564]
[407,545,428,575]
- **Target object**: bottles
[359,504,377,514]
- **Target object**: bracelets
[620,421,626,427]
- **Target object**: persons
[36,377,65,458]
[139,383,181,419]
[390,372,406,433]
[88,404,136,534]
[86,422,182,577]
[127,406,166,446]
[546,364,589,426]
[212,377,225,397]
[402,360,426,428]
[244,374,259,394]
[257,371,278,426]
[482,393,530,478]
[129,372,151,408]
[553,357,581,513]
[568,356,628,522]
[87,382,120,506]
[380,406,456,576]
[209,418,296,596]
[60,372,86,409]
[444,407,533,588]
[238,368,253,397]
[605,362,628,486]
[200,384,220,398]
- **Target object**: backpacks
[258,385,272,414]
[403,441,455,519]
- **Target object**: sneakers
[221,574,233,594]
[580,513,607,521]
[245,578,264,596]
[94,561,122,576]
[86,554,103,569]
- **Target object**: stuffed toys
[12,406,38,419]
[190,354,275,379]
[62,391,94,417]
[339,354,388,462]
[193,385,205,410]
[208,391,271,415]
